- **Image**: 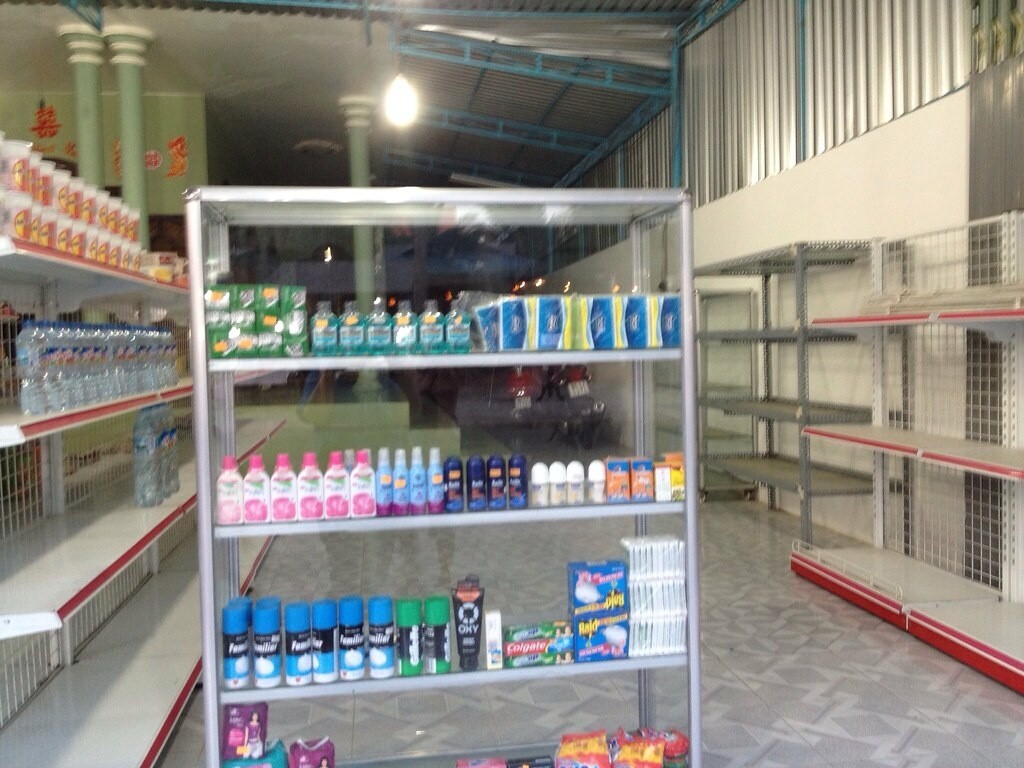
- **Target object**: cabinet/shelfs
[902,210,1024,700]
[181,187,703,768]
[791,211,1014,635]
[647,276,764,506]
[692,237,886,548]
[1,237,286,768]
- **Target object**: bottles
[13,320,180,415]
[133,403,180,508]
